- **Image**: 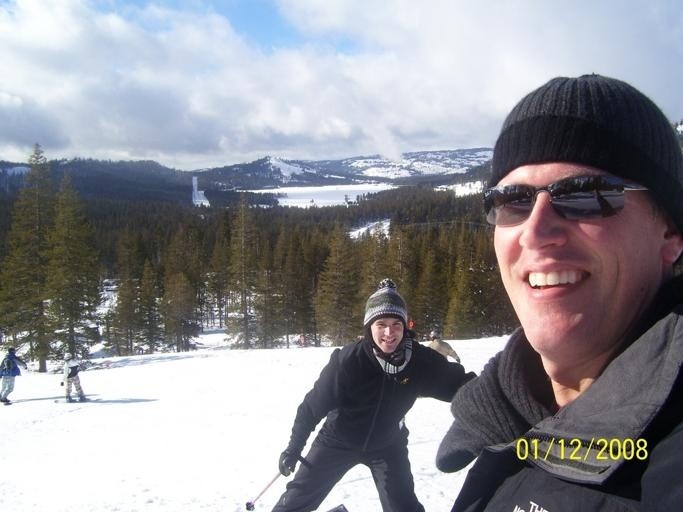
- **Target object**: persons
[0,346,28,404]
[426,330,460,363]
[58,352,86,402]
[264,275,481,512]
[436,72,683,511]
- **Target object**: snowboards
[1,397,11,406]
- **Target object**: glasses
[480,171,652,226]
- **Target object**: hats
[363,278,409,333]
[482,71,682,241]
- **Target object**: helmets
[429,331,440,341]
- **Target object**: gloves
[456,357,460,364]
[278,444,302,477]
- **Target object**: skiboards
[53,394,100,403]
